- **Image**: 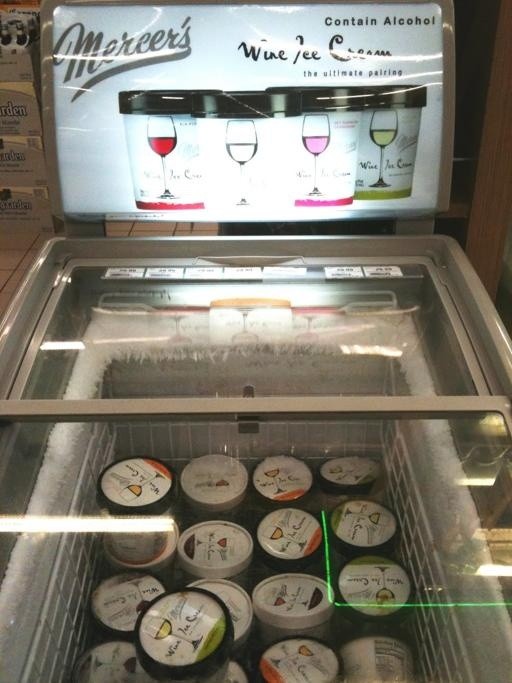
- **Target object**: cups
[134,587,236,682]
[96,454,178,564]
[176,520,255,588]
[88,571,166,638]
[190,91,303,213]
[340,635,415,683]
[118,89,223,210]
[319,457,383,502]
[70,639,136,683]
[252,453,317,509]
[256,635,344,683]
[249,572,336,647]
[178,578,253,661]
[258,509,324,575]
[180,453,250,519]
[266,86,361,207]
[324,501,402,565]
[353,85,427,200]
[336,554,412,636]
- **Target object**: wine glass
[215,471,230,492]
[196,538,233,548]
[301,114,330,197]
[369,110,398,188]
[146,116,178,200]
[127,580,151,615]
[224,120,258,205]
[328,465,362,481]
[374,566,396,605]
[264,596,309,608]
[119,472,165,503]
[232,307,259,345]
[263,464,288,495]
[296,315,319,344]
[167,315,192,343]
[269,642,317,668]
[262,525,307,552]
[144,616,204,653]
[344,507,390,527]
